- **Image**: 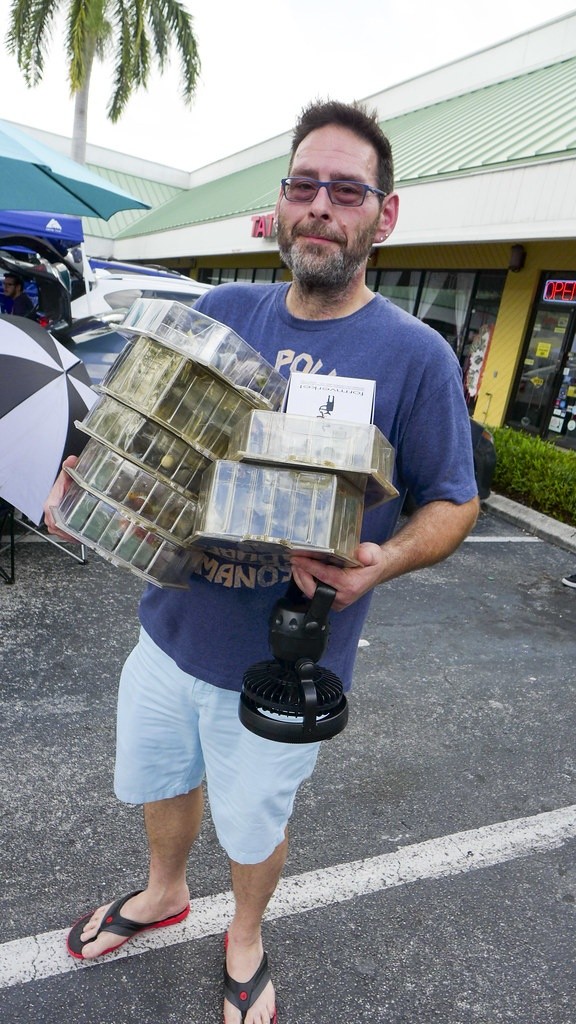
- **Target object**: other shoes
[562,574,576,588]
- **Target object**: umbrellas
[0,119,152,222]
[0,313,102,528]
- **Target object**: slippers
[219,932,277,1024]
[67,888,189,959]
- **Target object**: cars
[0,234,499,520]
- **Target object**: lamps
[508,243,527,272]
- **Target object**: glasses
[2,282,14,287]
[281,177,388,207]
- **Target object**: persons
[3,257,47,317]
[45,94,480,1024]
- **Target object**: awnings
[0,210,84,254]
